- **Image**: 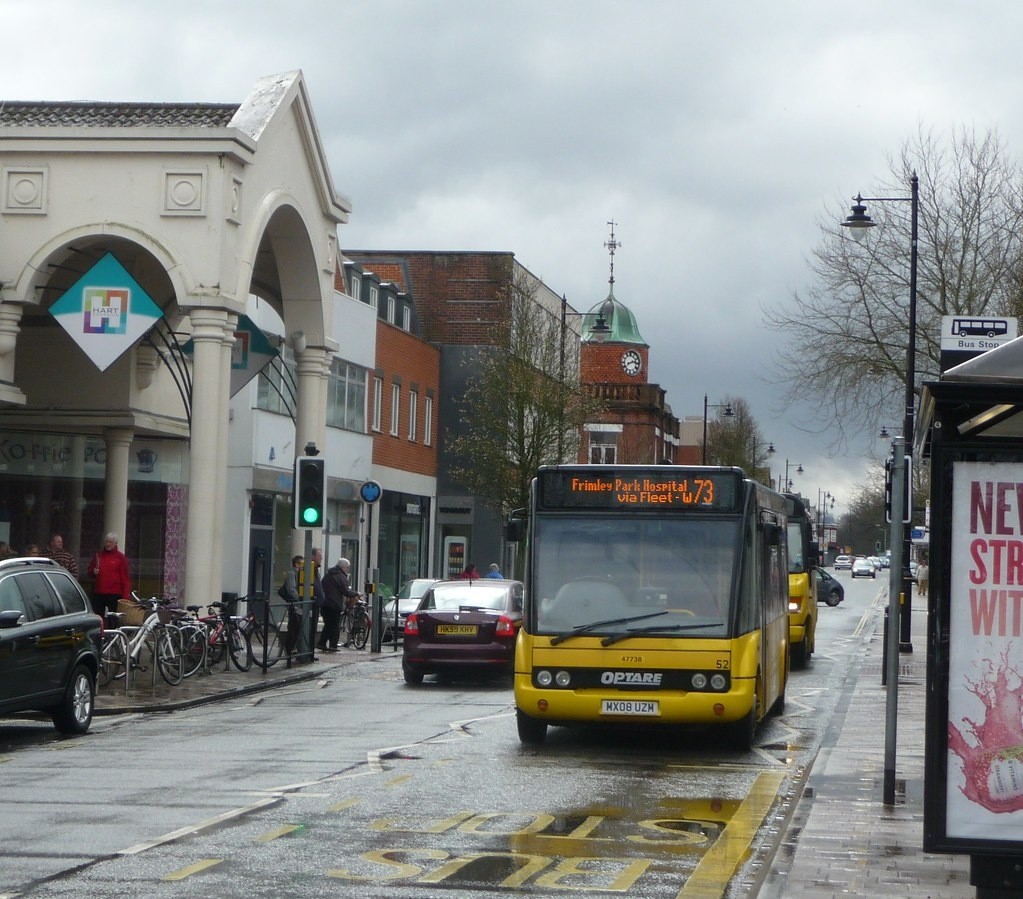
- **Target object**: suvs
[0,556,108,735]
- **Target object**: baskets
[116,599,145,625]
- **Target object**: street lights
[879,528,887,552]
[817,488,832,567]
[557,294,615,465]
[840,166,920,805]
[752,436,776,479]
[785,459,804,493]
[703,393,734,466]
[821,492,836,567]
[778,474,794,493]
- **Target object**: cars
[381,578,446,643]
[909,561,920,584]
[815,565,845,607]
[402,577,524,685]
[849,559,878,578]
[833,549,891,572]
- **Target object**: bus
[779,492,819,671]
[506,464,790,754]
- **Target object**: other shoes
[317,644,329,651]
[328,646,340,652]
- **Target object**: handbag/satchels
[277,582,292,603]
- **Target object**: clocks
[621,350,642,376]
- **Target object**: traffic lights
[875,542,881,552]
[295,456,327,530]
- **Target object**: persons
[297,548,325,661]
[915,560,928,596]
[27,544,40,557]
[38,534,78,582]
[285,555,303,650]
[88,531,131,618]
[0,541,18,560]
[318,558,363,651]
[486,564,503,579]
[462,564,479,578]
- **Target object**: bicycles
[95,586,372,688]
